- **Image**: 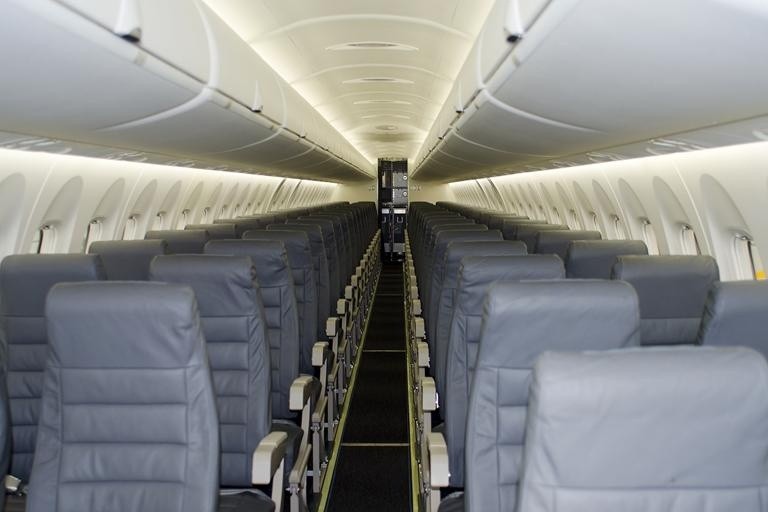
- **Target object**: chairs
[408,197,768,507]
[2,200,381,512]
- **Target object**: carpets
[322,249,415,507]
